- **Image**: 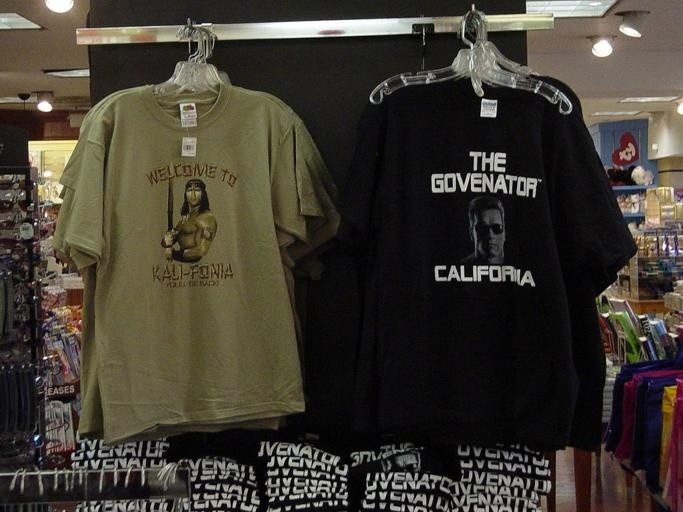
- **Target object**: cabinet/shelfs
[599,183,683,335]
[1,165,81,475]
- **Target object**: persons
[462,195,518,268]
[161,178,217,263]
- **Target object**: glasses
[473,224,502,235]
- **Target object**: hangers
[154,12,248,112]
[368,12,573,116]
[0,461,193,512]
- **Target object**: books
[600,295,679,362]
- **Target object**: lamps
[36,92,53,113]
[587,11,650,60]
